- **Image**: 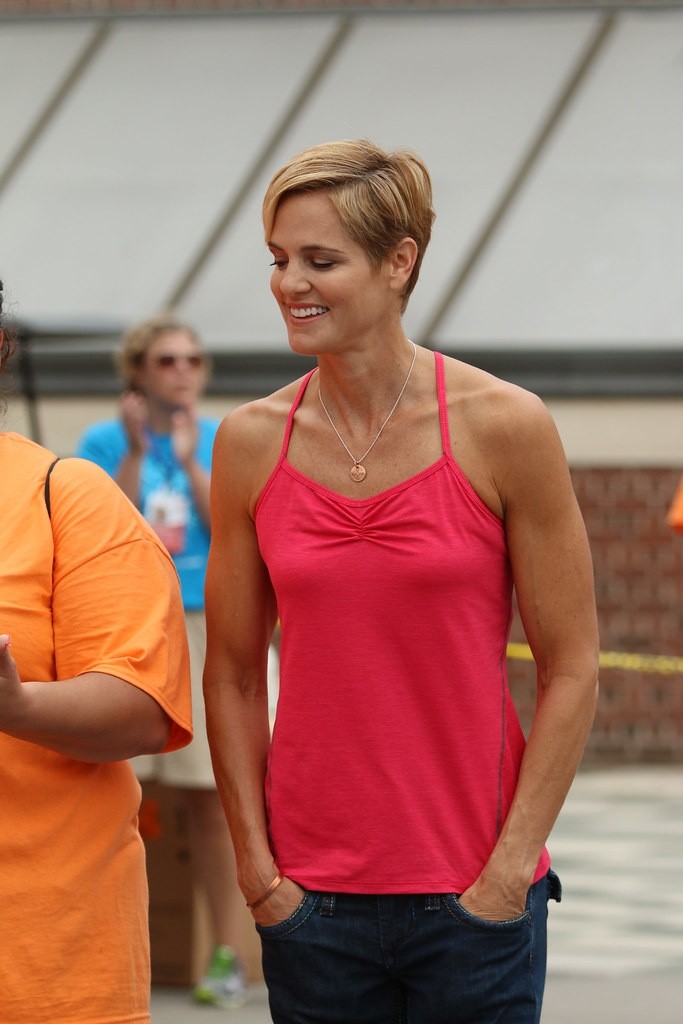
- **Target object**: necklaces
[320,338,416,482]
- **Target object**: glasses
[158,355,204,368]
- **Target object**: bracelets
[247,872,284,910]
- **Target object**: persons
[76,321,242,1006]
[202,141,599,1024]
[0,281,193,1024]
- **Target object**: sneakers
[191,944,250,1007]
